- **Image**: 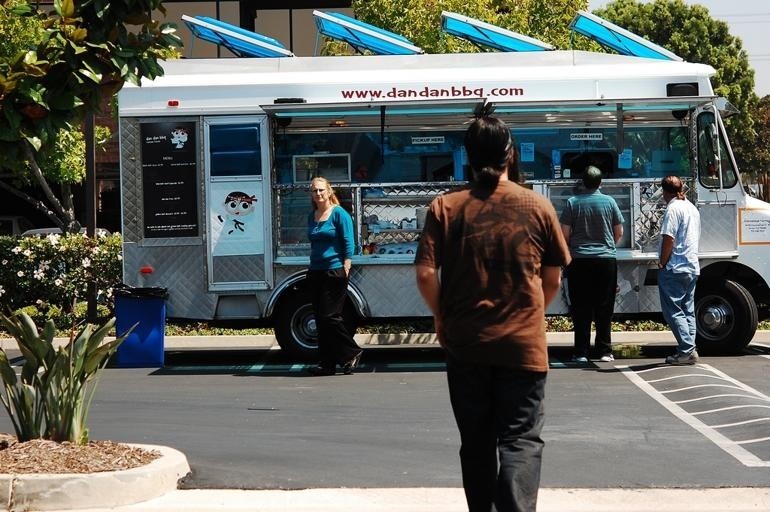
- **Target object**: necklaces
[314,205,333,226]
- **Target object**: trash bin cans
[115,288,166,368]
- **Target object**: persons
[341,161,386,200]
[558,165,626,364]
[413,116,572,512]
[656,175,701,364]
[307,177,365,376]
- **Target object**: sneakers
[600,354,614,362]
[666,350,699,364]
[572,356,590,362]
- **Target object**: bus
[119,10,770,365]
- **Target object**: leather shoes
[344,352,361,373]
[310,365,336,376]
